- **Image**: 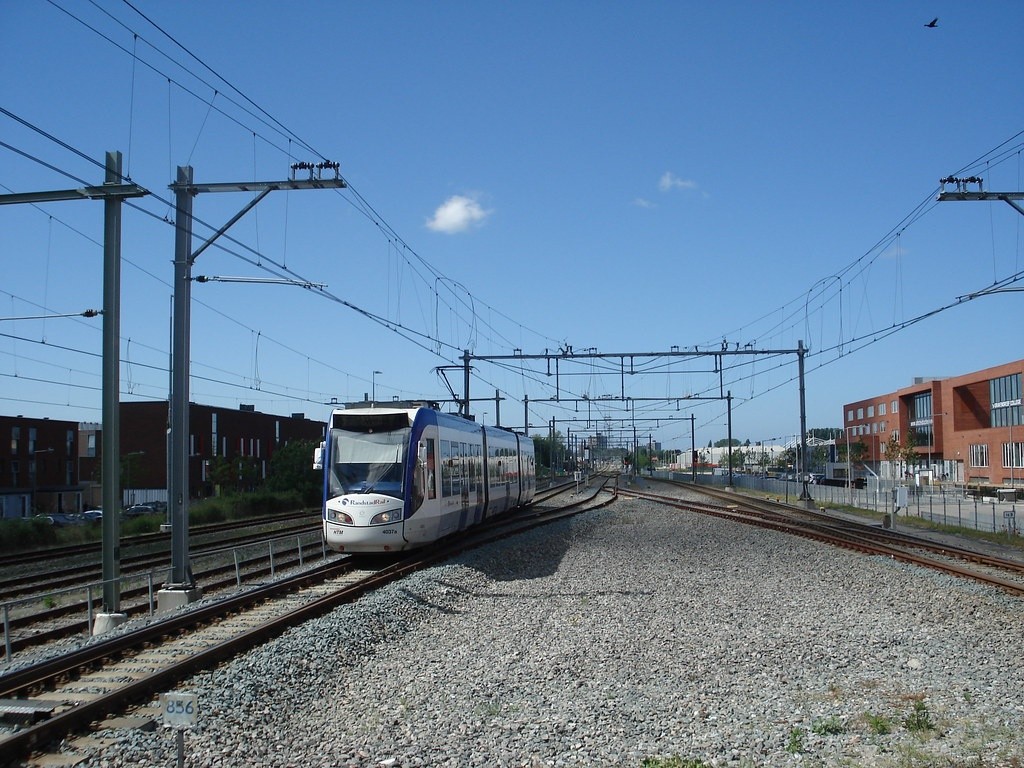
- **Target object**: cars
[766,465,836,487]
[21,500,166,532]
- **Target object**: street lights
[846,423,869,503]
[928,412,946,470]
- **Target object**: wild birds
[924,16,939,28]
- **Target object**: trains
[308,398,538,564]
